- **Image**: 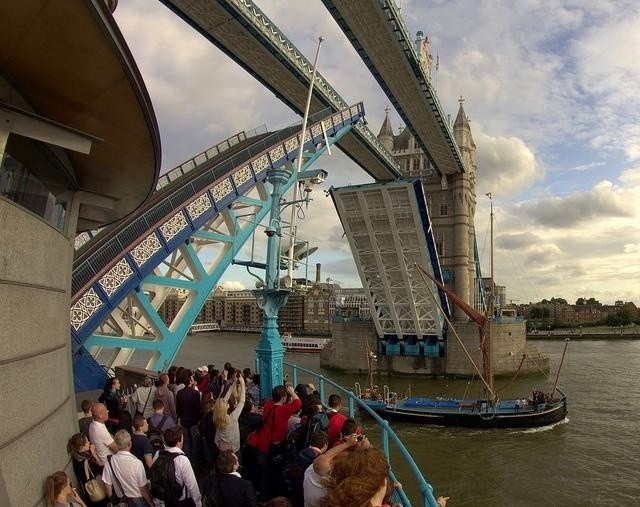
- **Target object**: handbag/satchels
[190,425,202,443]
[135,406,143,417]
[246,431,272,453]
[83,458,107,502]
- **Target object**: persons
[521,398,526,410]
[515,397,520,412]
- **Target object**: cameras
[355,435,365,442]
[287,391,298,399]
[236,374,240,379]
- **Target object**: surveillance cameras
[264,226,277,237]
[297,169,328,183]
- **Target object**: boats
[280,331,334,352]
[349,189,571,427]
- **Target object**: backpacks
[145,415,168,451]
[148,450,186,503]
[284,425,304,464]
[305,414,324,446]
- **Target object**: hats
[208,365,214,369]
[198,365,209,373]
